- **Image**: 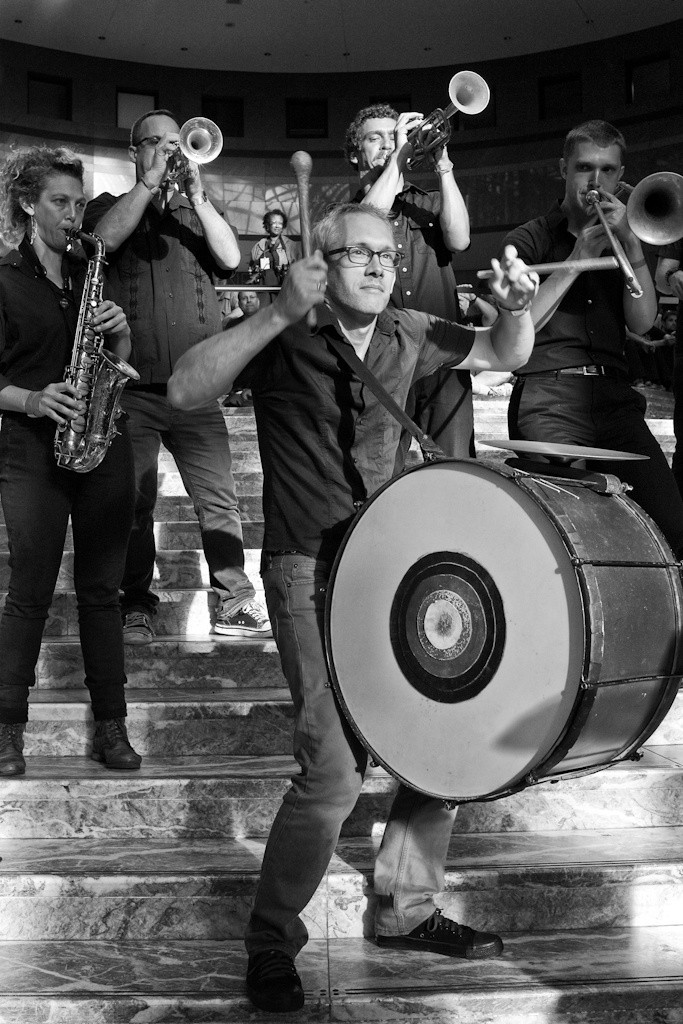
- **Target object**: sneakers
[376,907,504,959]
[91,717,142,769]
[245,949,304,1013]
[0,721,26,776]
[214,598,273,637]
[122,612,156,645]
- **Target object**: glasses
[323,246,405,268]
[132,137,159,146]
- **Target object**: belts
[551,364,625,378]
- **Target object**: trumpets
[407,70,490,166]
[165,116,223,182]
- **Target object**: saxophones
[50,228,142,475]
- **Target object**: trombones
[585,172,682,298]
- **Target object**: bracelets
[666,267,680,286]
[434,160,453,177]
[630,258,647,270]
[189,190,207,207]
[140,173,161,201]
[25,391,40,418]
[496,299,531,317]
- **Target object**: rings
[318,281,321,291]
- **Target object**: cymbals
[481,438,654,462]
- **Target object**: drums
[321,455,683,806]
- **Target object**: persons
[625,309,677,388]
[347,104,477,462]
[500,122,683,563]
[167,204,539,1011]
[215,209,299,333]
[0,143,144,779]
[80,110,276,644]
[654,237,683,487]
